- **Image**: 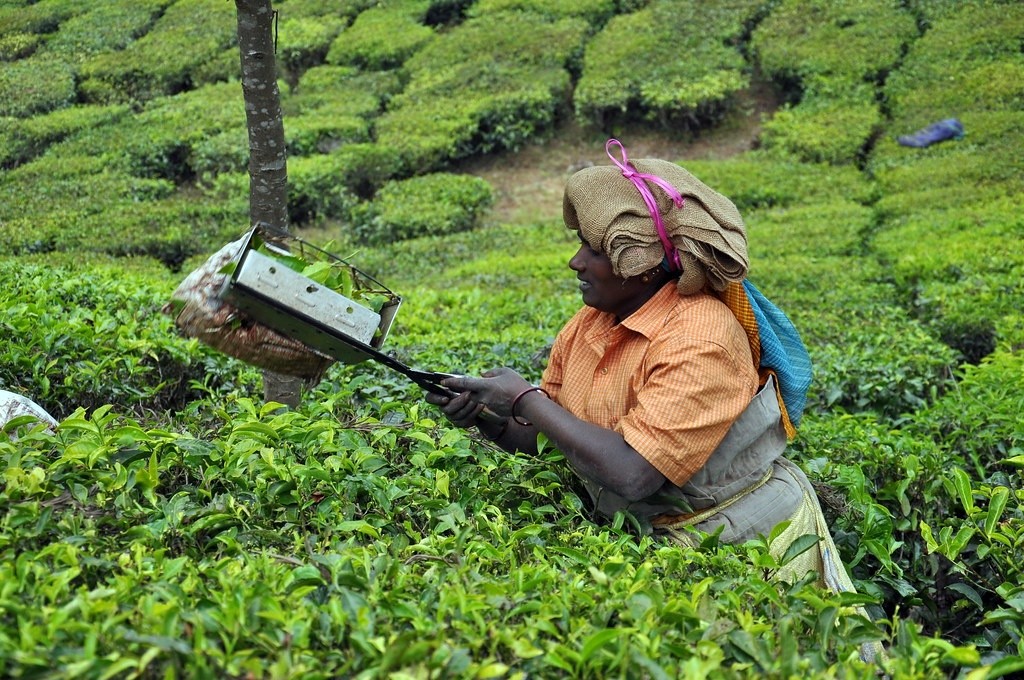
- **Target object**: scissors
[233,283,489,426]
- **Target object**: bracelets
[512,387,550,426]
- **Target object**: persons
[424,139,891,669]
